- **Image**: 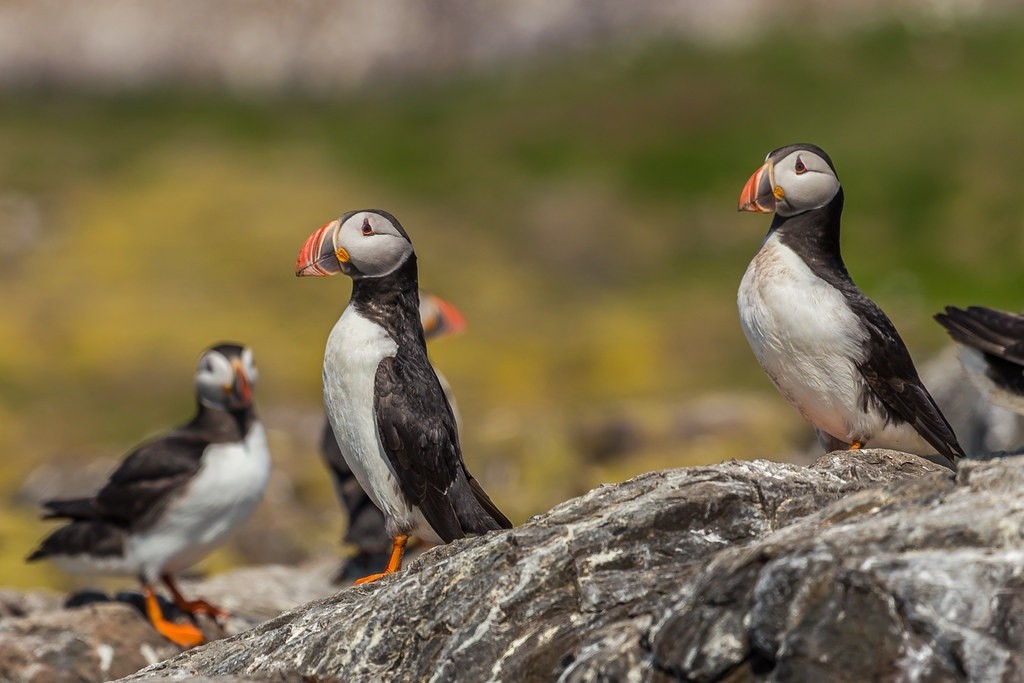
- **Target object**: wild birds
[293,210,509,586]
[735,142,1024,468]
[21,341,272,652]
